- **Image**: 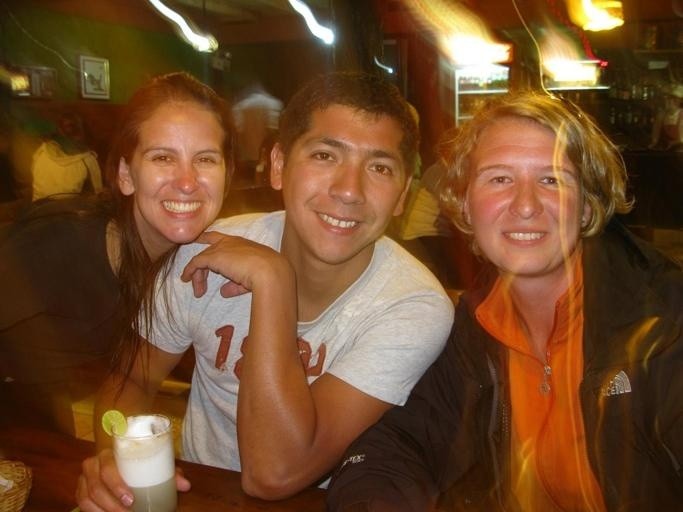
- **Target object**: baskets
[1,459,34,511]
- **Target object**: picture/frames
[79,54,110,99]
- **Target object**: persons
[31,108,104,199]
[227,73,283,184]
[385,152,420,239]
[77,73,454,512]
[0,75,234,476]
[326,95,682,512]
[647,83,683,152]
[80,111,120,199]
[401,128,463,289]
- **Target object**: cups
[114,413,178,512]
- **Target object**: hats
[670,83,682,98]
